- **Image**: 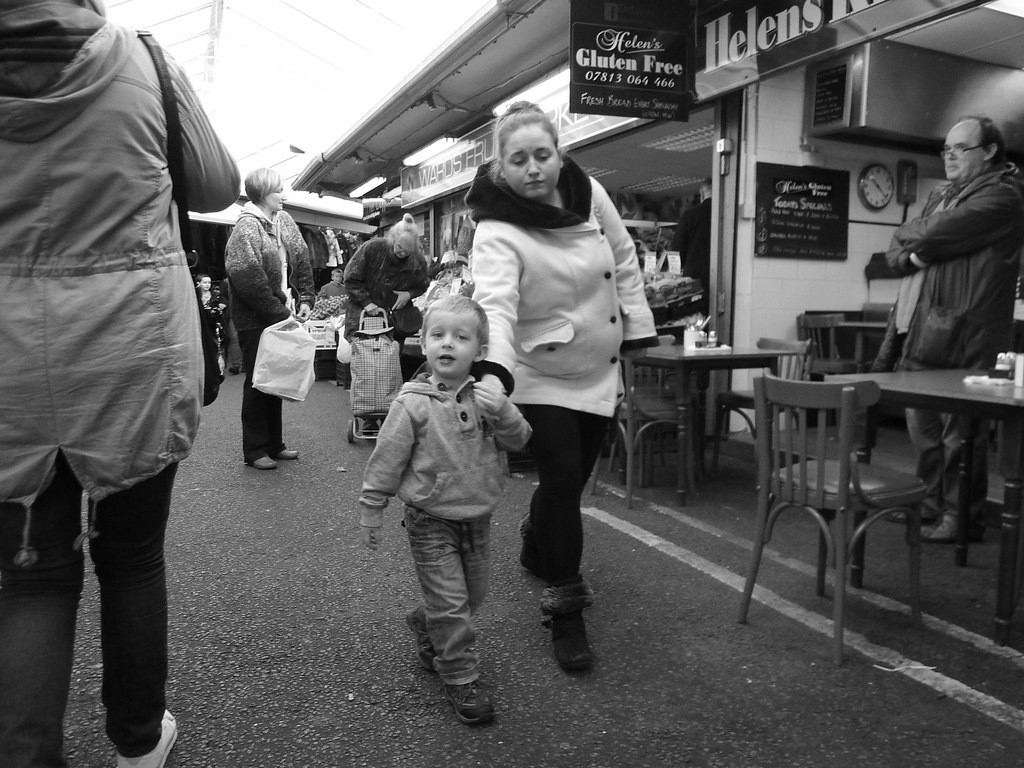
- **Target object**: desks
[824,368,1024,647]
[624,346,797,507]
[838,321,890,373]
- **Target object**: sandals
[884,499,941,525]
[920,512,986,542]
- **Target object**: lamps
[348,170,389,198]
[491,60,570,117]
[402,132,458,167]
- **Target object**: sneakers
[405,605,436,671]
[116,708,177,768]
[441,679,495,723]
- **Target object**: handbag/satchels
[456,212,474,255]
[186,249,224,408]
[389,308,423,336]
[252,314,316,402]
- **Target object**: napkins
[692,344,732,356]
[963,375,1015,387]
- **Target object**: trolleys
[346,306,403,444]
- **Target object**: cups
[683,330,703,348]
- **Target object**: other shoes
[275,449,298,459]
[253,457,276,468]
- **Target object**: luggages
[347,308,404,444]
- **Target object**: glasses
[939,143,989,160]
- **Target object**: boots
[520,513,549,577]
[539,575,595,669]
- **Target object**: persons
[359,293,533,725]
[317,269,348,303]
[225,168,315,470]
[0,0,241,767]
[195,274,230,356]
[669,176,712,328]
[463,99,662,672]
[344,213,430,435]
[884,114,1024,542]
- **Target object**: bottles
[698,331,718,345]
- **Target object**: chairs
[588,303,929,666]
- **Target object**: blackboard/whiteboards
[754,162,848,260]
[809,54,854,134]
[569,0,699,122]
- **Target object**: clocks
[857,163,894,210]
